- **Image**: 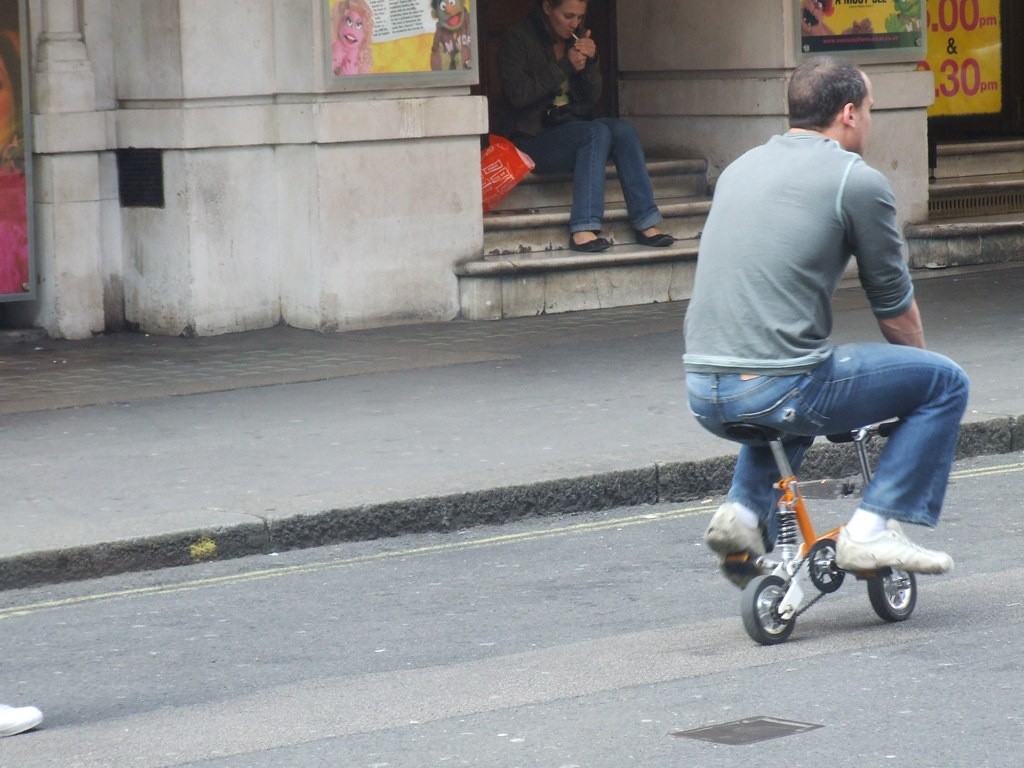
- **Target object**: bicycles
[725,421,917,645]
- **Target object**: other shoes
[637,231,674,247]
[570,236,610,252]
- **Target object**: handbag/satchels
[481,134,536,214]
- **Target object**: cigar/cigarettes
[571,32,579,40]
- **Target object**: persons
[687,59,968,589]
[501,0,673,251]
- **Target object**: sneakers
[0,704,43,737]
[703,502,765,589]
[835,519,954,575]
